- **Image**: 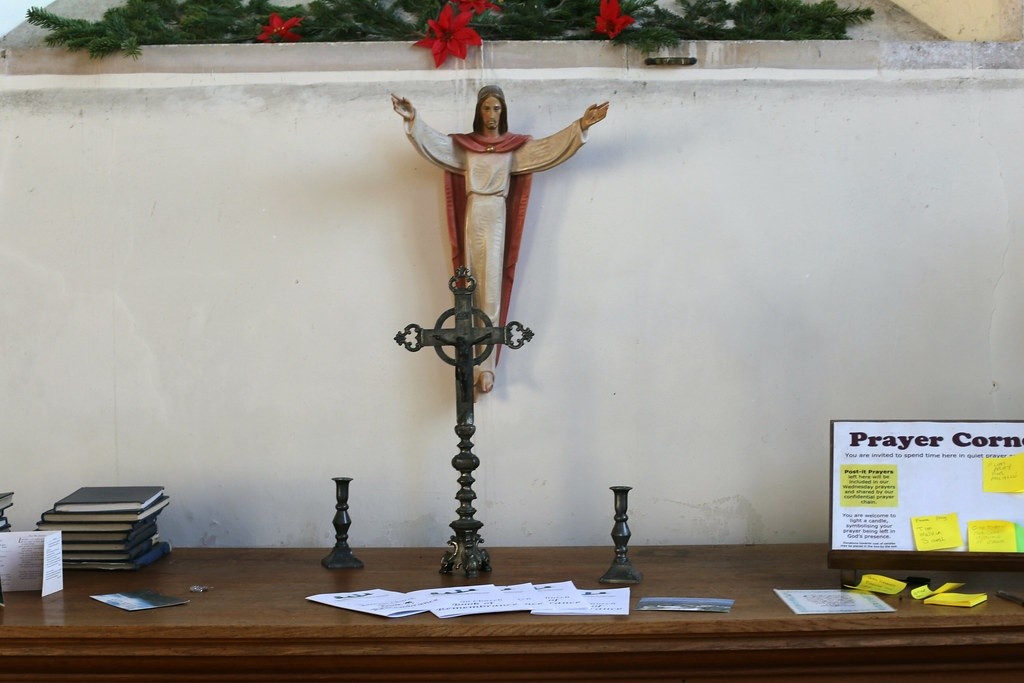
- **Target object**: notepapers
[924,592,987,608]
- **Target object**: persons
[387,83,610,405]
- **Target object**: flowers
[258,0,636,70]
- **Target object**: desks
[1,543,1024,682]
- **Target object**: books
[0,492,16,532]
[33,484,174,571]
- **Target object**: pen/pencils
[995,591,1024,607]
